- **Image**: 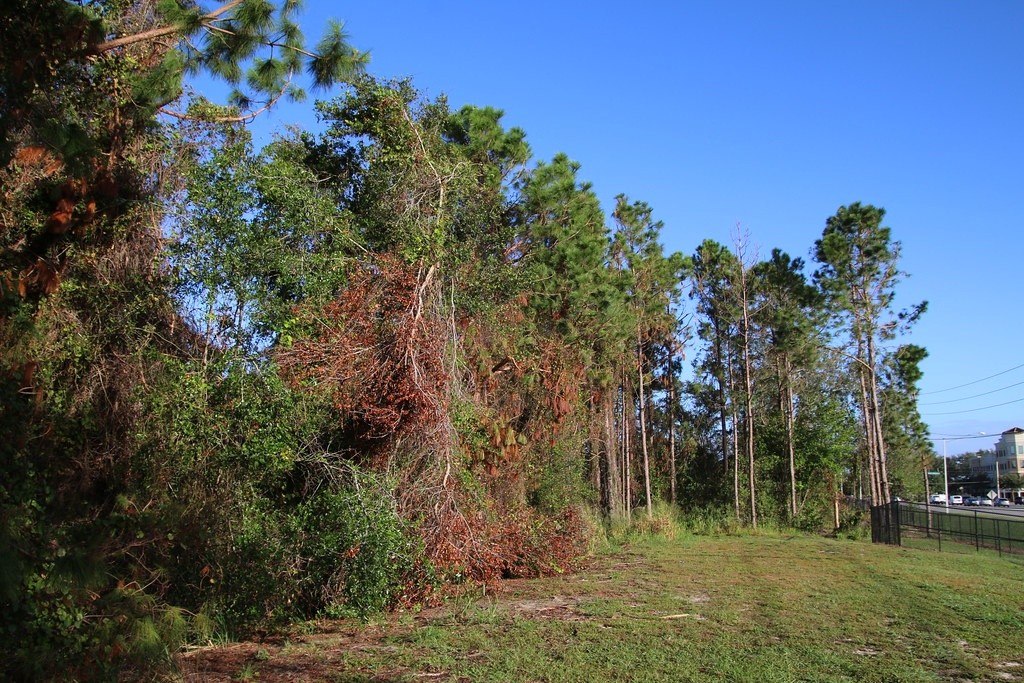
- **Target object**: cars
[993,497,1009,507]
[1015,496,1024,505]
[931,492,992,506]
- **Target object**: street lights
[942,431,987,514]
